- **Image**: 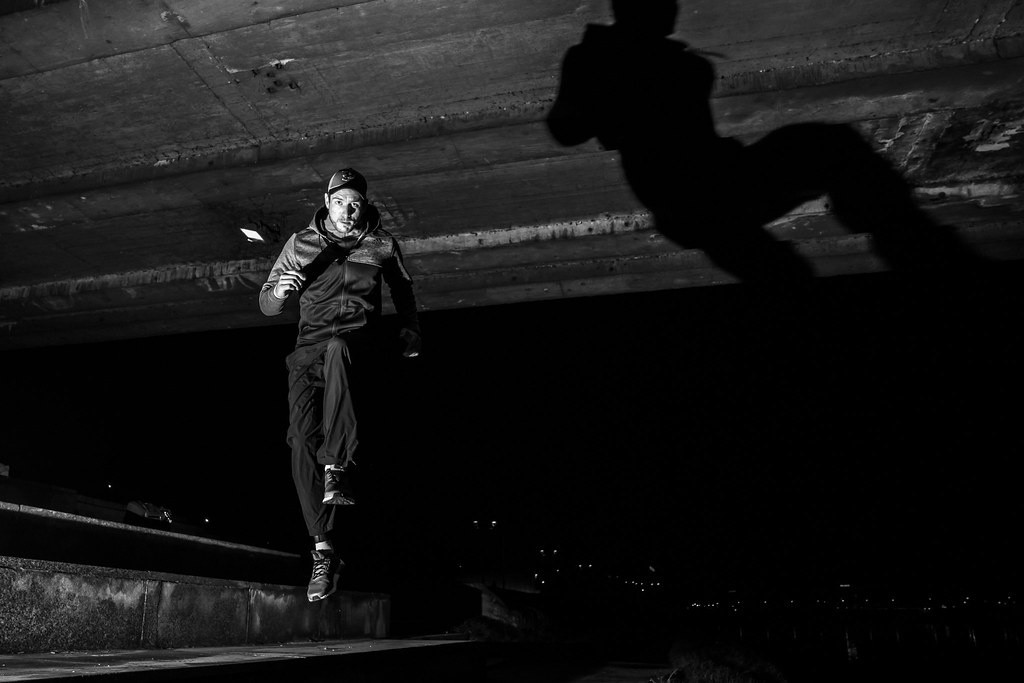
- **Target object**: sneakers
[307,544,345,602]
[322,468,355,505]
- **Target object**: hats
[327,168,367,201]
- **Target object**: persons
[258,167,423,603]
